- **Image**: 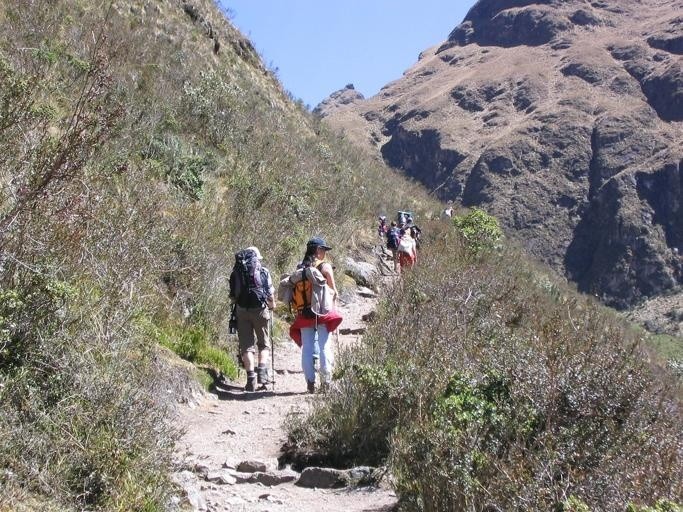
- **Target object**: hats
[307,237,333,251]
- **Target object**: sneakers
[257,373,277,384]
[241,381,256,392]
[307,380,319,394]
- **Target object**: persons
[227,246,275,393]
[294,237,338,393]
[376,210,420,274]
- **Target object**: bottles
[227,313,238,335]
[283,317,298,327]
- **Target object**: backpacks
[230,249,269,310]
[290,279,319,319]
[387,230,418,259]
